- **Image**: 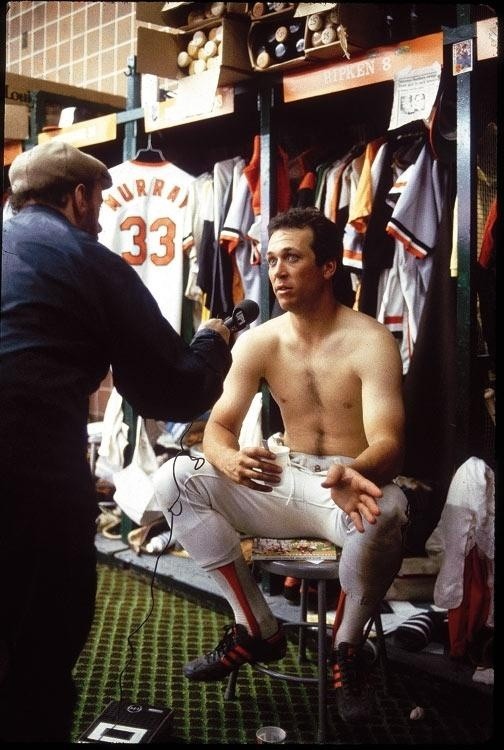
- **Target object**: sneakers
[330,641,372,725]
[183,621,287,683]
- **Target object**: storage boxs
[135,0,225,31]
[245,10,309,72]
[137,21,247,118]
[5,71,127,128]
[293,0,376,62]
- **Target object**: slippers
[392,612,436,653]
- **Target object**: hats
[8,139,112,196]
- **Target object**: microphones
[225,300,260,334]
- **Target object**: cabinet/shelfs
[255,11,462,671]
[126,51,263,614]
[32,91,126,562]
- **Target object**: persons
[461,44,470,68]
[151,208,411,727]
[1,139,231,750]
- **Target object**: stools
[221,556,389,743]
